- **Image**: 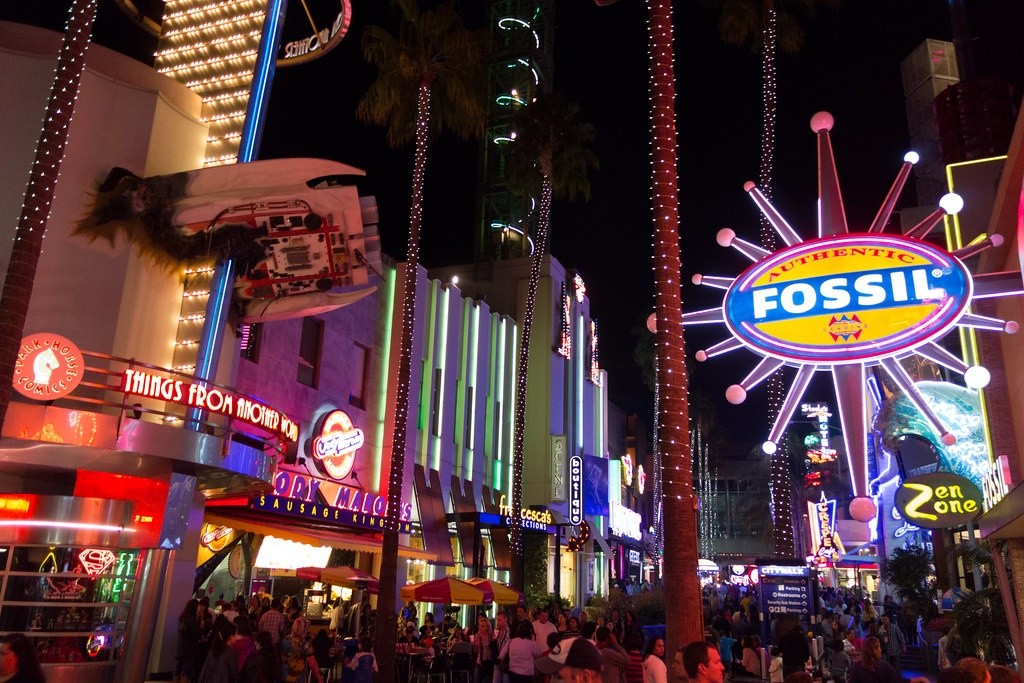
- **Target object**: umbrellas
[400,576,494,651]
[463,577,523,625]
[297,565,379,649]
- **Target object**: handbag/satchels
[178,619,201,641]
[498,641,511,675]
[493,649,501,665]
[480,660,494,675]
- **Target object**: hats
[456,628,466,636]
[534,635,605,674]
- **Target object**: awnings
[201,509,437,561]
[589,521,614,559]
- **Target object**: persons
[174,595,381,683]
[0,633,45,683]
[672,582,992,683]
[399,602,668,683]
[612,578,664,597]
